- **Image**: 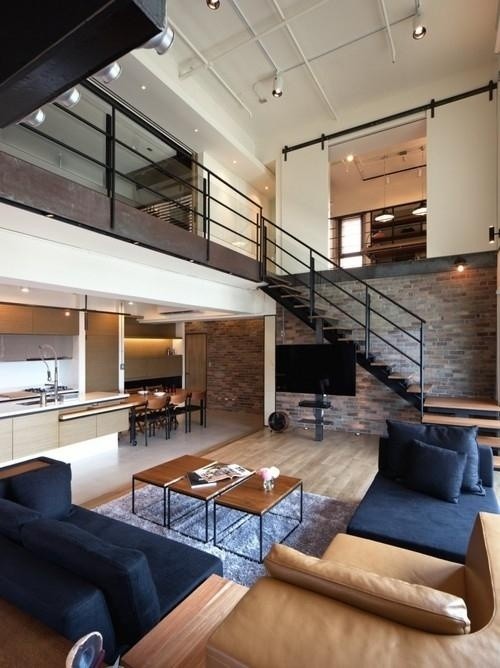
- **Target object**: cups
[170,384,176,392]
[163,384,169,393]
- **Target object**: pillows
[383,417,487,506]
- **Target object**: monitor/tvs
[274,342,357,407]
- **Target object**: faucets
[38,344,62,398]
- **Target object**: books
[186,462,252,489]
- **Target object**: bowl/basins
[154,392,166,397]
[138,390,149,395]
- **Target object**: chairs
[129,387,208,447]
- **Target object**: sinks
[17,396,57,407]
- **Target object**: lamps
[207,1,220,13]
[271,74,284,101]
[413,8,428,42]
[373,154,395,223]
[451,259,469,274]
[488,225,497,243]
[412,145,429,217]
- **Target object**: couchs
[0,456,223,654]
[346,444,499,564]
[210,513,499,666]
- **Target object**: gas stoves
[25,384,74,394]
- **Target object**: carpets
[91,485,358,587]
